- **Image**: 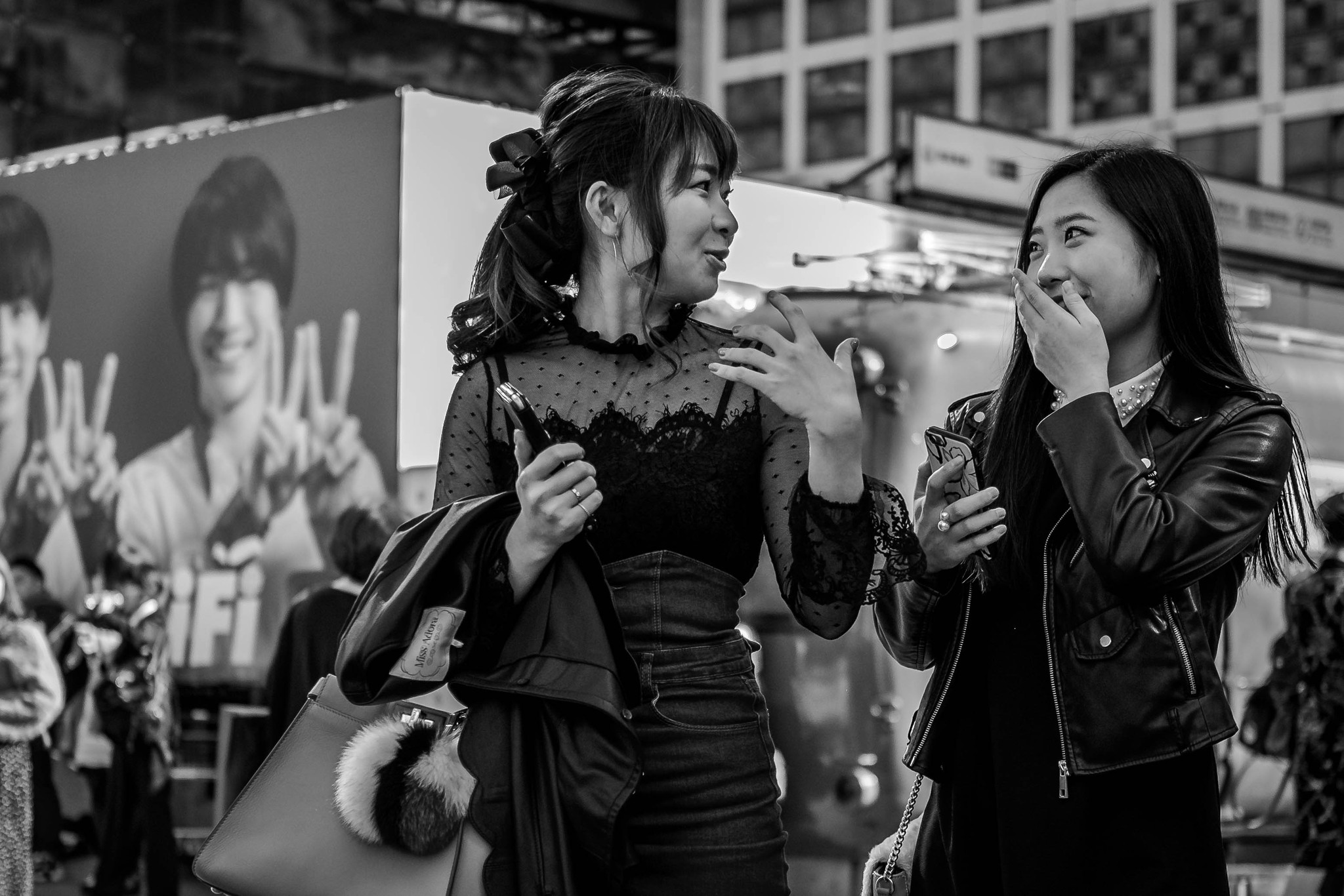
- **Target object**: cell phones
[923,424,996,567]
[493,382,601,535]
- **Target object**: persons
[113,153,390,668]
[1274,493,1344,896]
[0,540,184,896]
[264,497,415,744]
[0,192,123,573]
[430,65,929,896]
[872,144,1329,896]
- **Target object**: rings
[569,487,582,503]
[578,503,591,517]
[938,505,955,532]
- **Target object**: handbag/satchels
[1238,678,1295,760]
[862,810,922,896]
[193,674,493,896]
